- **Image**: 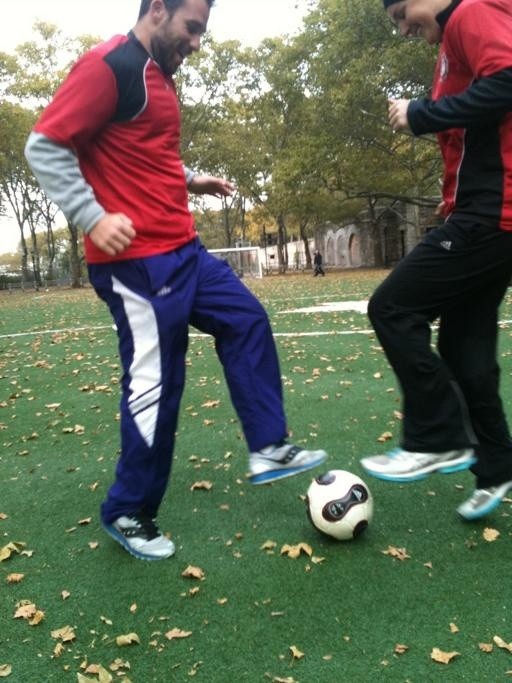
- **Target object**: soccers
[305,469,374,542]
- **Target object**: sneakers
[103,514,177,562]
[455,479,512,521]
[246,442,329,486]
[357,444,479,484]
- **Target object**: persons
[26,0,330,560]
[313,250,324,276]
[358,0,512,522]
